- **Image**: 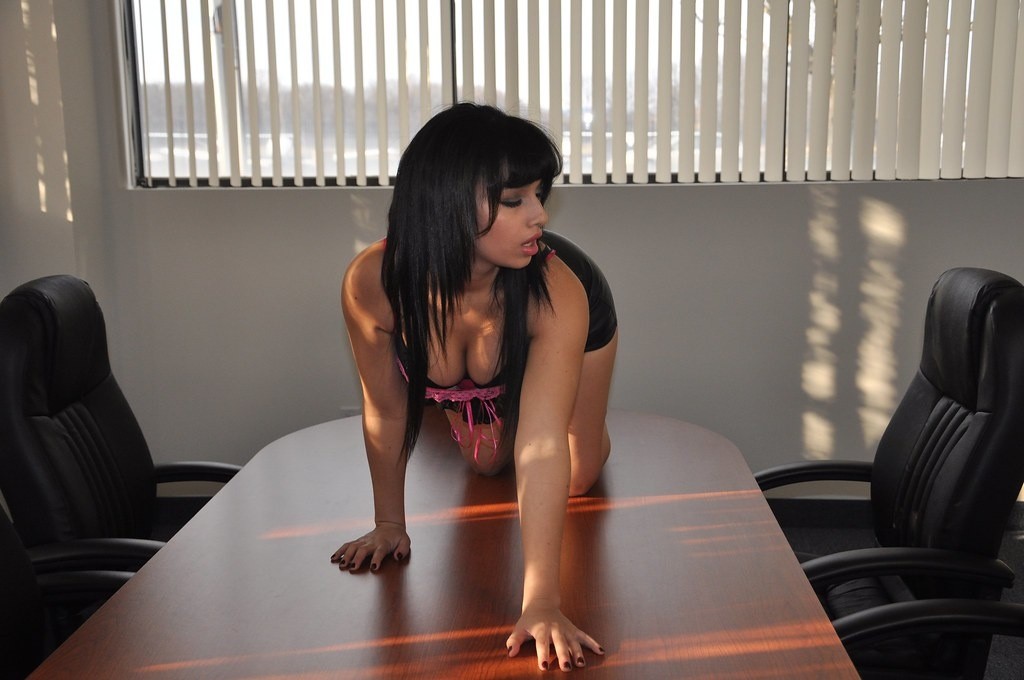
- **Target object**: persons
[322,100,619,675]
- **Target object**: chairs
[0,500,135,680]
[0,275,246,571]
[752,266,1023,679]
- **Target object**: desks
[26,403,864,680]
